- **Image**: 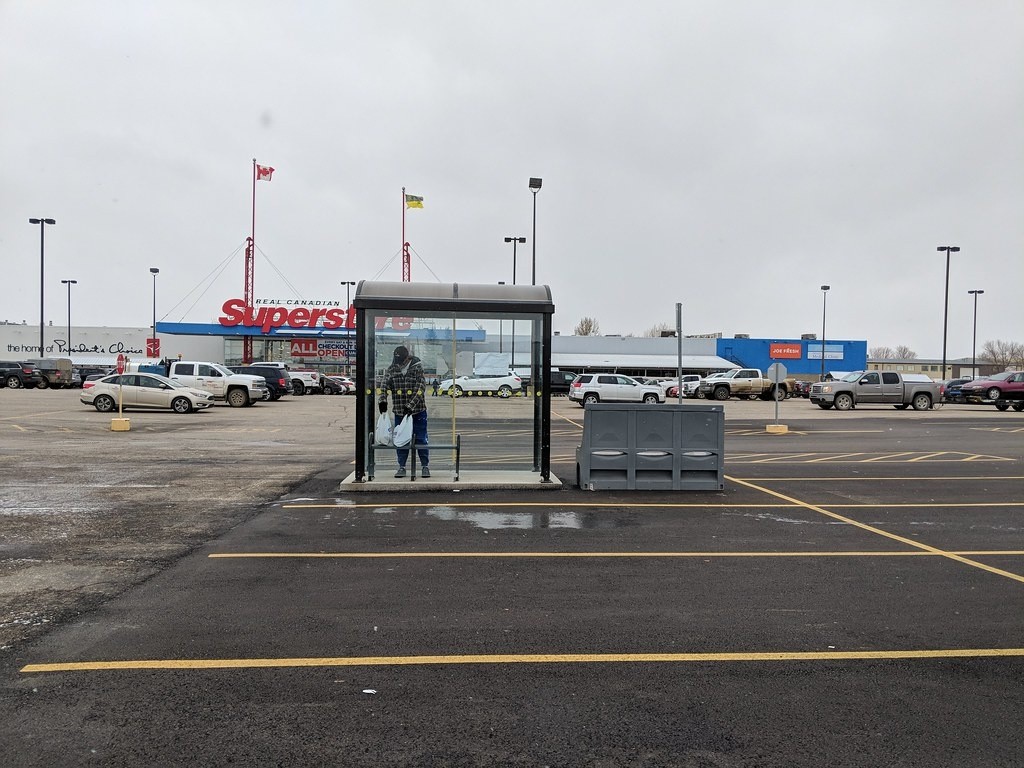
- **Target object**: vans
[80,367,116,387]
[222,365,295,401]
[26,358,73,389]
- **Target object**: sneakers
[394,467,406,477]
[422,467,431,477]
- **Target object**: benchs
[576,403,725,491]
[368,431,460,482]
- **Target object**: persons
[432,379,440,396]
[378,346,430,478]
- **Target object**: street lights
[936,246,960,380]
[341,281,355,374]
[29,218,55,357]
[504,236,526,371]
[967,289,984,381]
[820,285,830,381]
[150,267,159,358]
[529,177,542,378]
[61,280,77,356]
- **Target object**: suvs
[437,371,523,398]
[525,370,727,408]
[0,360,43,388]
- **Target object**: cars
[79,372,216,413]
[933,370,1024,401]
[793,380,812,398]
[72,369,83,387]
[321,374,356,395]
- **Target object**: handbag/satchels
[374,411,392,446]
[393,414,413,448]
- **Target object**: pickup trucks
[249,362,321,396]
[700,368,796,401]
[809,370,940,411]
[167,362,271,407]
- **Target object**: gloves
[403,408,412,416]
[379,402,387,414]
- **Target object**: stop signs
[117,354,125,374]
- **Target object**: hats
[394,346,408,364]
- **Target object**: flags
[405,194,423,210]
[256,165,274,181]
